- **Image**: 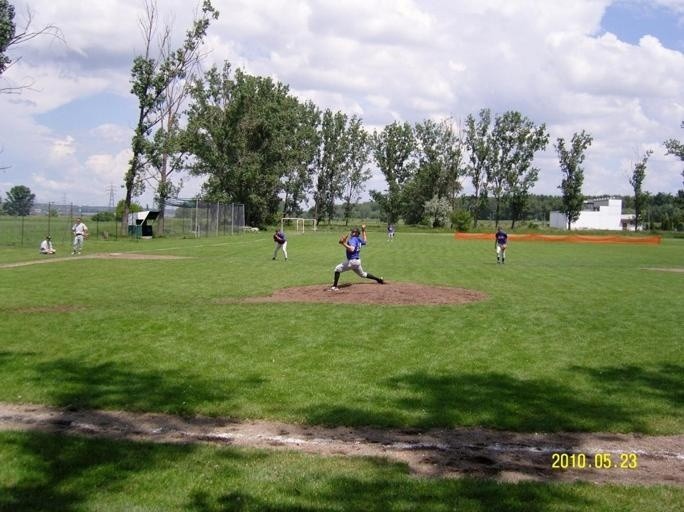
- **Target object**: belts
[76,234,82,235]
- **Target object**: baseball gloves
[339,233,348,244]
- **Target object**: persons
[494,226,508,265]
[386,223,394,241]
[270,228,288,260]
[39,235,56,254]
[324,223,383,292]
[69,218,88,256]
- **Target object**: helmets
[351,228,361,234]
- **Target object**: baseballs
[362,224,366,228]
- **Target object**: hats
[47,235,52,239]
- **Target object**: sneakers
[328,286,340,292]
